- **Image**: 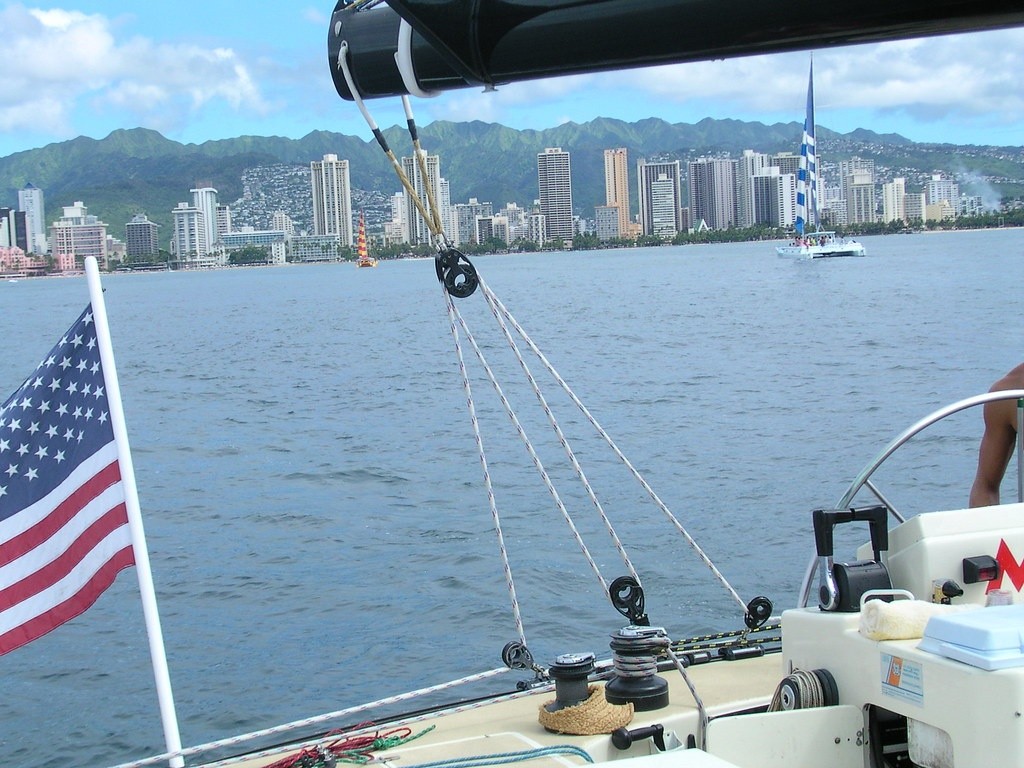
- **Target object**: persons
[969,362,1024,507]
[790,235,833,247]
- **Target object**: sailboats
[358,207,377,268]
[775,51,867,258]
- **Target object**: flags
[0,304,136,661]
